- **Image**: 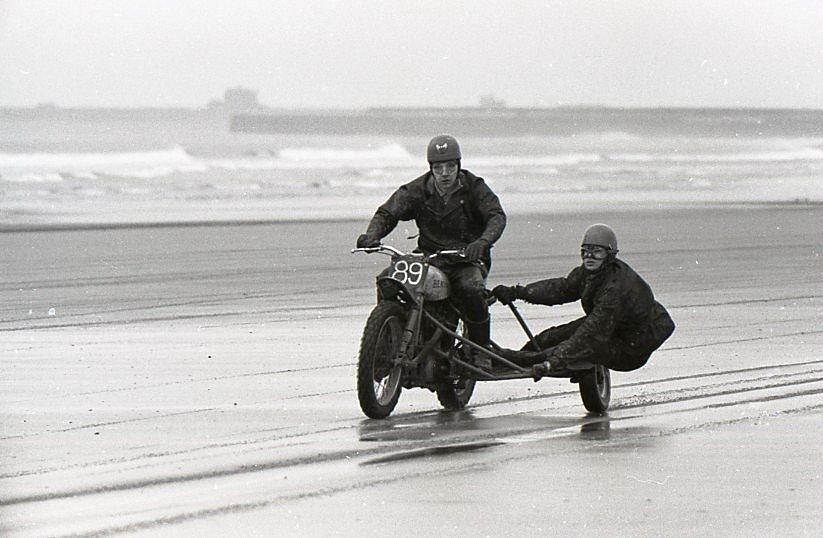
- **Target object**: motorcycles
[350,243,611,419]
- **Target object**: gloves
[357,228,383,254]
[533,363,548,382]
[465,239,490,263]
[492,285,516,305]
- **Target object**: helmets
[427,135,461,162]
[581,223,619,254]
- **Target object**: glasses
[581,247,609,259]
[431,160,459,174]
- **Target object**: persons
[488,224,676,382]
[356,135,506,372]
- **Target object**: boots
[465,312,492,370]
